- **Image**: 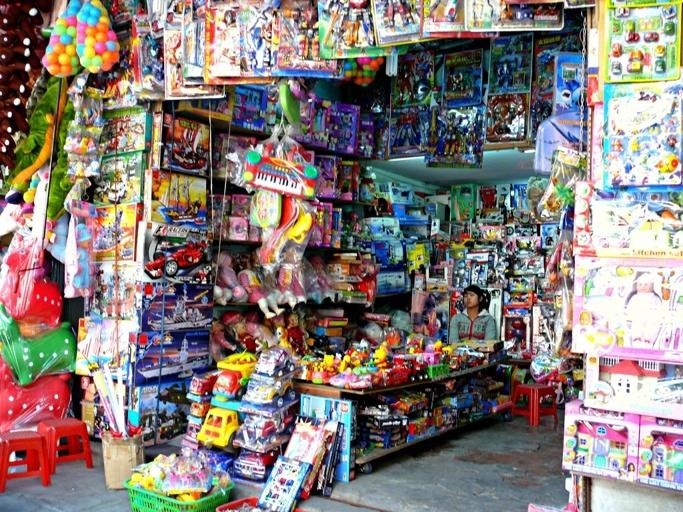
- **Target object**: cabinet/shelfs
[204,120,413,371]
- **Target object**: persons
[211,312,317,360]
[625,272,665,348]
[1,203,45,316]
[215,251,339,318]
[448,285,496,344]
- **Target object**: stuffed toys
[76,217,94,249]
[0,1,94,254]
[45,214,69,264]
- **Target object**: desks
[288,359,501,475]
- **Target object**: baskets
[216,495,307,512]
[123,478,236,512]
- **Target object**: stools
[0,415,94,492]
[509,382,558,429]
